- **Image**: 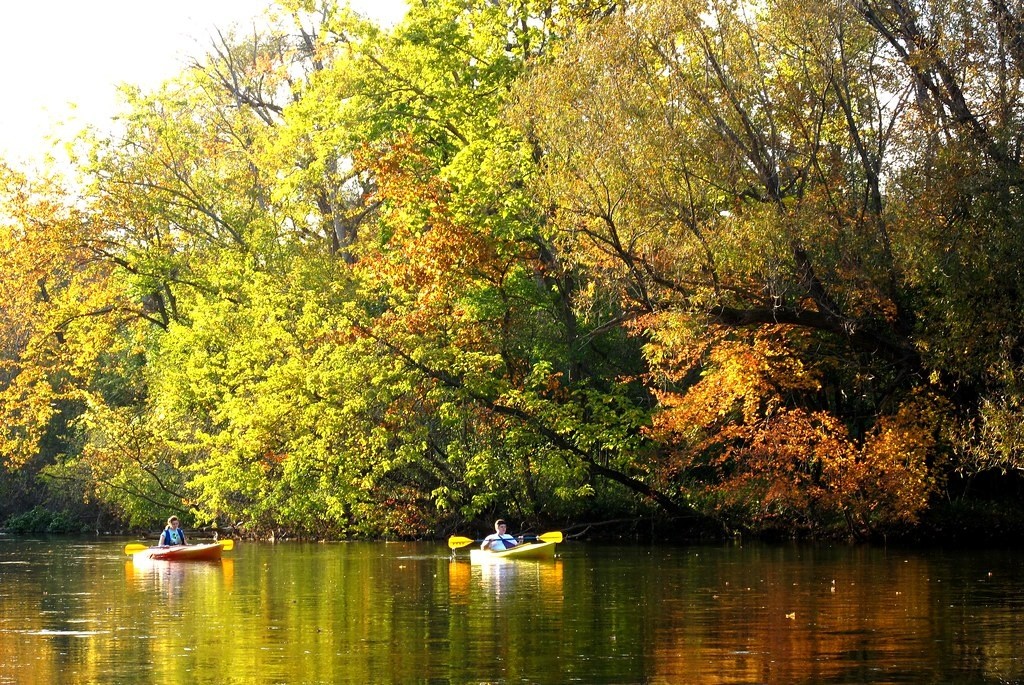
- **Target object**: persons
[158,516,188,546]
[481,519,523,551]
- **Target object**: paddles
[125,539,234,555]
[448,531,563,549]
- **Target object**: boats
[134,542,224,562]
[467,539,556,567]
